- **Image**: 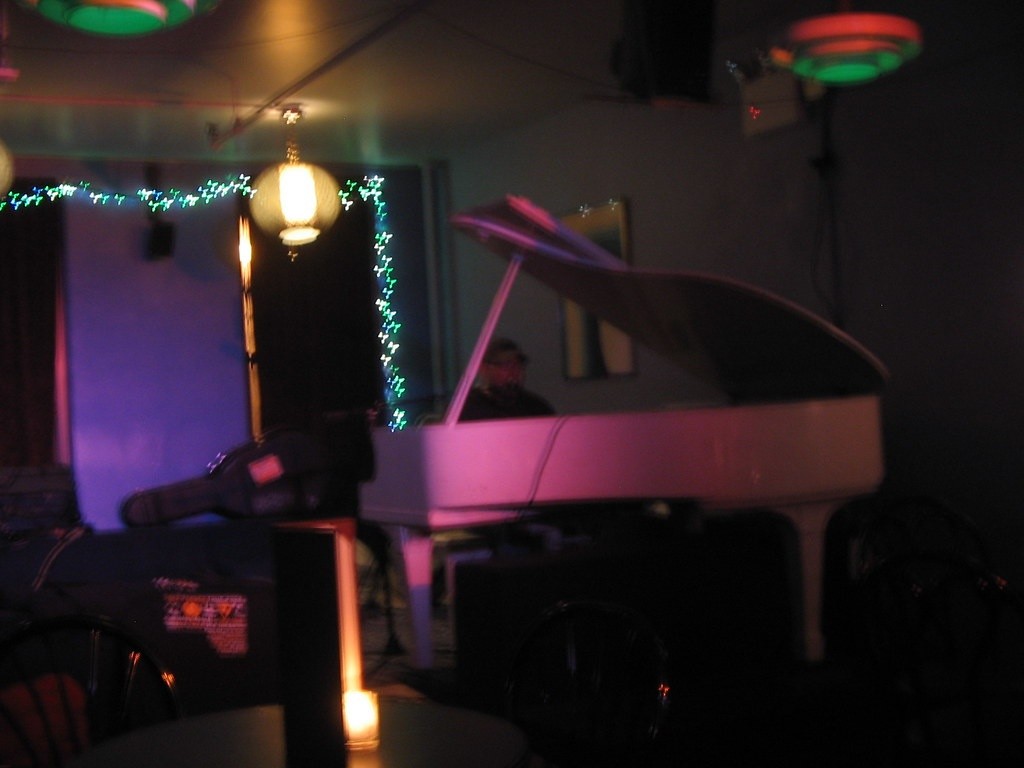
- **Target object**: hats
[484,338,530,368]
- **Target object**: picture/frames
[557,193,638,383]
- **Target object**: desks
[65,692,535,768]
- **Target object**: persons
[461,340,557,421]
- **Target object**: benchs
[387,504,670,566]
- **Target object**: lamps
[248,107,343,263]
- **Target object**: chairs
[0,609,184,768]
[858,494,1024,768]
[500,597,662,768]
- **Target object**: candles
[342,690,380,751]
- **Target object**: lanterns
[248,164,339,246]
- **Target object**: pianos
[357,193,894,680]
